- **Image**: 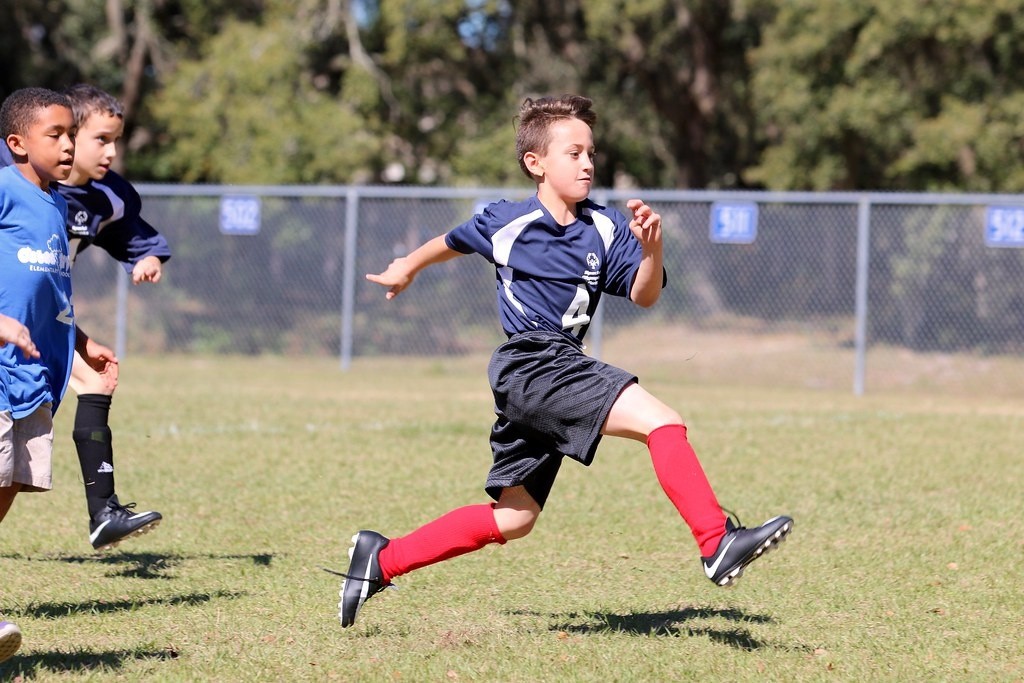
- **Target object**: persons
[1,86,119,668]
[1,82,172,552]
[337,96,795,630]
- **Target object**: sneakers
[0,621,22,664]
[89,494,162,552]
[700,506,794,587]
[316,529,397,628]
